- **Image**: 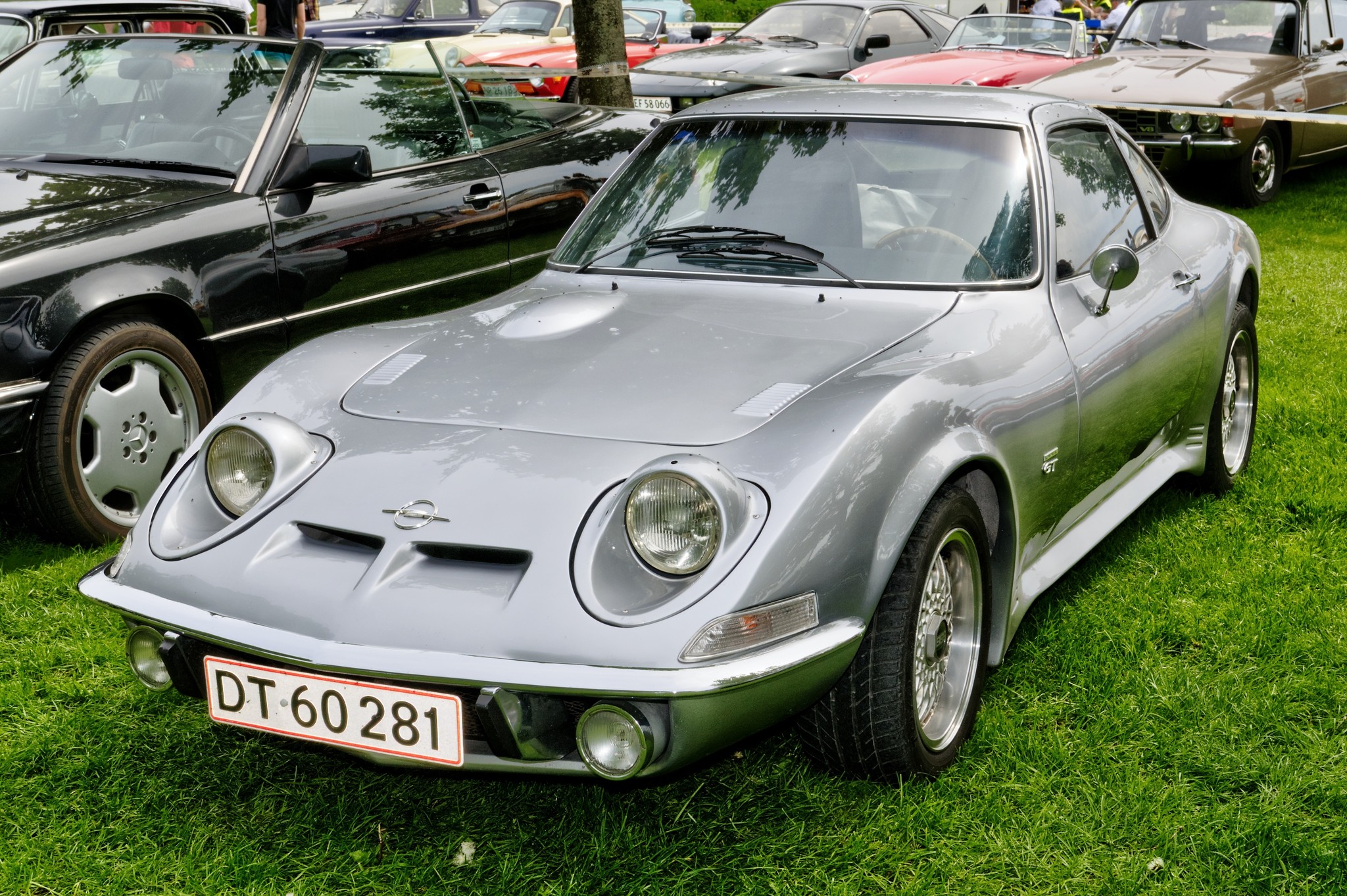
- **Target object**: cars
[1011,0,1347,212]
[0,0,507,104]
[629,0,1004,114]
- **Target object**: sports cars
[72,85,1263,795]
[0,32,671,546]
[839,14,1109,88]
[376,0,759,104]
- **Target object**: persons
[1026,0,1213,48]
[140,0,306,43]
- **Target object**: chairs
[820,16,846,38]
[707,142,862,271]
[161,74,223,126]
[925,152,1030,277]
